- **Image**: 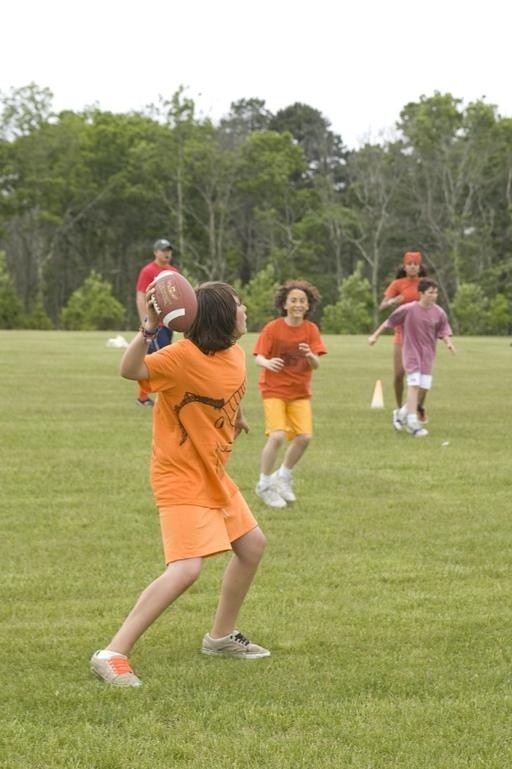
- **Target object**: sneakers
[405,424,429,438]
[135,397,154,409]
[391,409,404,432]
[89,648,143,689]
[268,471,296,503]
[254,484,287,509]
[417,405,429,426]
[201,630,271,660]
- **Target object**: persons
[378,252,431,424]
[134,238,177,410]
[253,278,327,509]
[85,268,271,688]
[368,280,456,438]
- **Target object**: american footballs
[151,270,198,332]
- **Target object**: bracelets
[138,325,159,344]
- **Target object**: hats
[153,237,175,251]
[402,251,422,266]
